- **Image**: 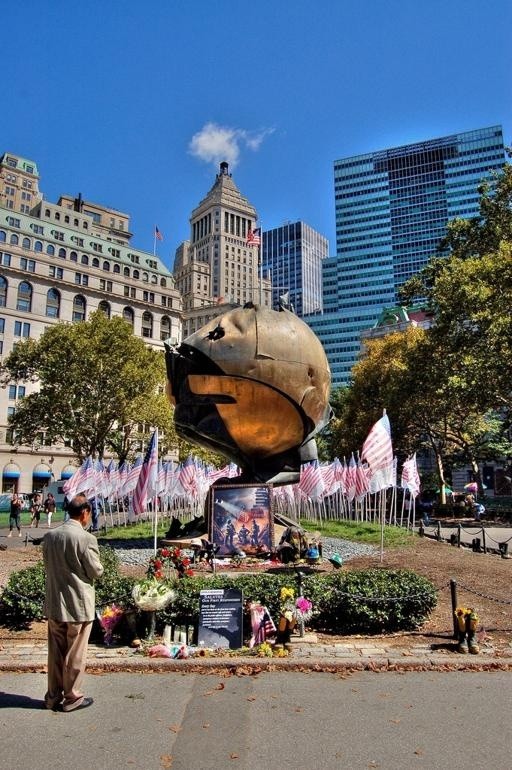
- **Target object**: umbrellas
[436,488,452,493]
[464,481,487,501]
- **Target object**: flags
[255,613,276,646]
[156,226,164,240]
[248,228,262,246]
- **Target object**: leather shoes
[77,698,93,709]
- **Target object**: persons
[43,493,56,527]
[31,494,41,528]
[41,496,105,711]
[7,493,22,537]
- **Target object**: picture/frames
[206,482,275,560]
